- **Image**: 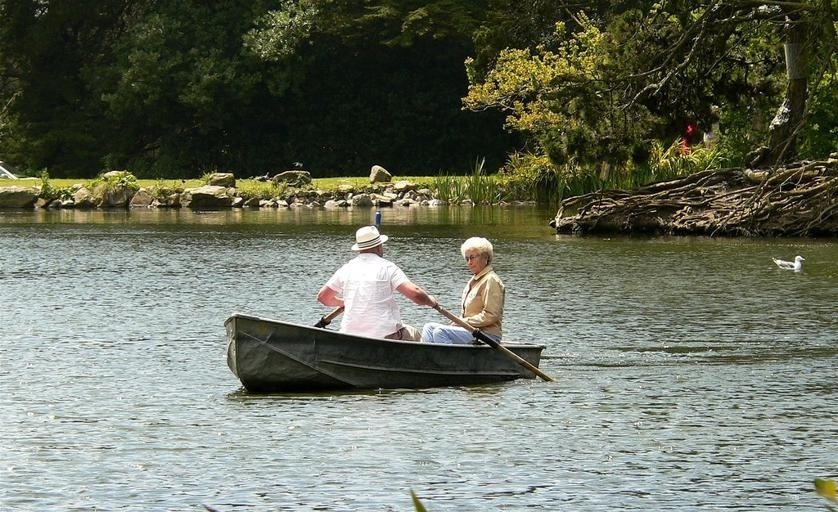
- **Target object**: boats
[221,313,547,394]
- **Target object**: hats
[351,227,388,251]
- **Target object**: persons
[420,236,506,343]
[317,225,437,342]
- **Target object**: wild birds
[772,256,805,270]
